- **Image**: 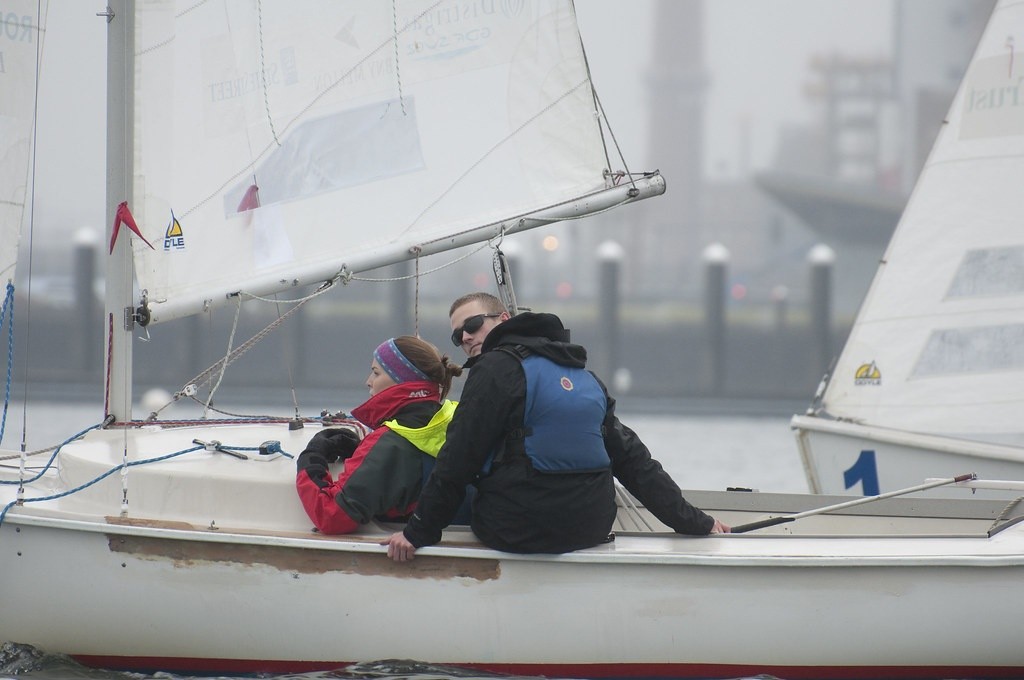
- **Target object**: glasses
[451,313,501,347]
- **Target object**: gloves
[305,428,344,463]
[331,428,361,461]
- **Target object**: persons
[295,335,461,536]
[377,291,731,563]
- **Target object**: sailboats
[0,0,1023,679]
[792,1,1024,498]
